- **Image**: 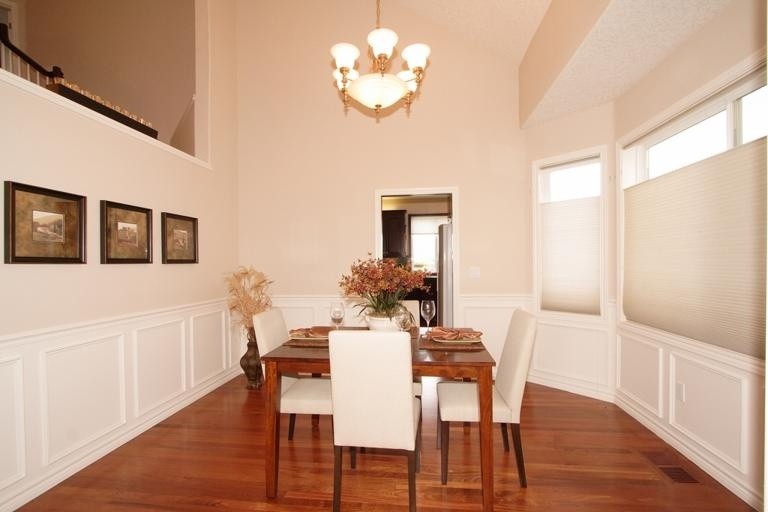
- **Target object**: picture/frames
[4,178,88,265]
[100,198,153,264]
[163,211,199,264]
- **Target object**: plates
[417,327,432,335]
[432,339,480,344]
[291,336,328,341]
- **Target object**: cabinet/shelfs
[382,209,411,266]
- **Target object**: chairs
[249,305,360,471]
[435,305,540,487]
[396,299,420,327]
[322,326,429,512]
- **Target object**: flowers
[336,253,430,317]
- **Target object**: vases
[240,329,262,386]
[364,314,406,331]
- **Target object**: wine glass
[329,301,344,330]
[420,300,435,338]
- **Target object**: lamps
[326,0,431,117]
